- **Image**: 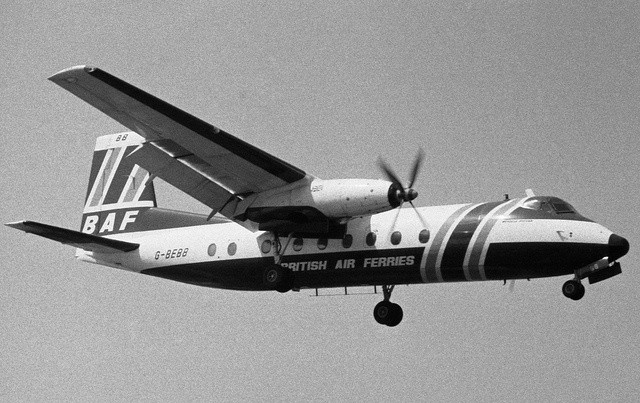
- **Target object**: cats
[4,64,630,327]
